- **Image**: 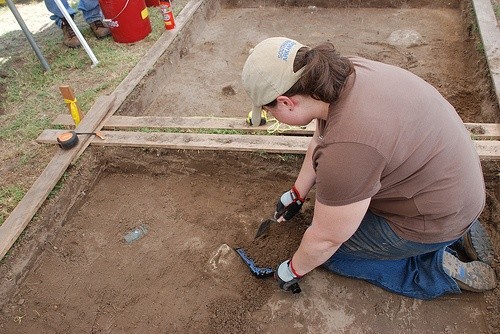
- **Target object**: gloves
[273,259,304,292]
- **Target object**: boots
[61,14,81,47]
[89,20,111,39]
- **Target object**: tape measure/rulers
[57,130,101,151]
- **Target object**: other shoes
[459,217,494,266]
[441,251,497,292]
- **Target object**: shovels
[255,219,281,237]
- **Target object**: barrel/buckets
[99,0,151,43]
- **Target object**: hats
[241,37,312,127]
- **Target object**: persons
[241,37,500,301]
[44,0,111,47]
